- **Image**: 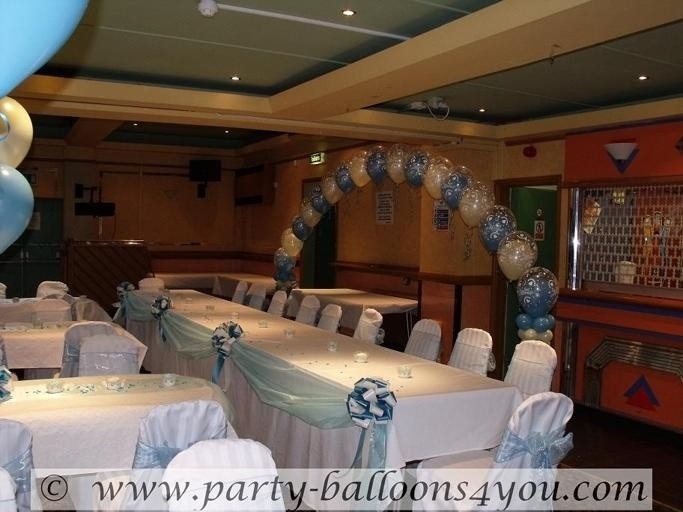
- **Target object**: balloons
[0,0,88,253]
[273,142,561,343]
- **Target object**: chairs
[404,320,577,512]
[231,282,383,344]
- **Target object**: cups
[184,297,191,302]
[231,311,239,319]
[104,376,120,389]
[161,372,176,388]
[353,351,369,363]
[46,380,63,393]
[257,319,268,329]
[206,305,214,312]
[397,365,411,378]
[283,328,295,339]
[326,340,338,352]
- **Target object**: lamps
[602,138,640,167]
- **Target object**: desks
[215,272,275,300]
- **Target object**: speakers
[74,202,116,217]
[188,159,222,182]
